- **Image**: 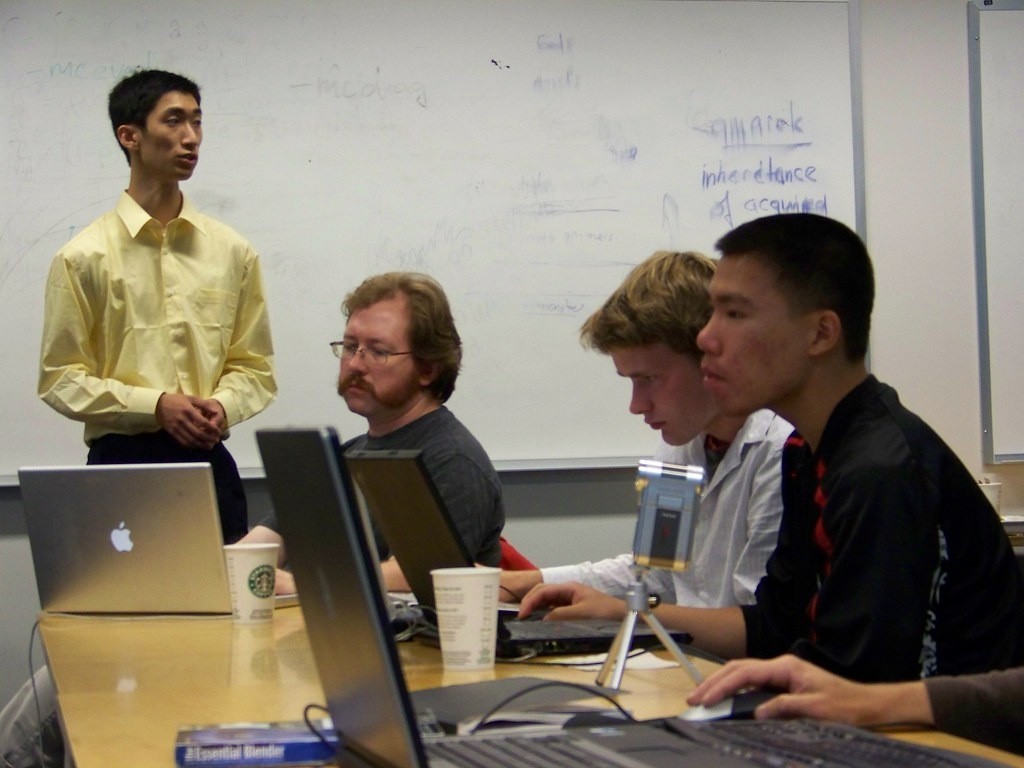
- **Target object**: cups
[223,543,279,624]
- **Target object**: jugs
[430,568,502,672]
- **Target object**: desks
[36,604,1023,768]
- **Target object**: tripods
[595,564,706,694]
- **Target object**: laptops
[16,426,1018,768]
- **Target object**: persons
[38,70,276,545]
[687,654,1024,755]
[476,250,791,607]
[233,273,507,591]
[515,212,1024,683]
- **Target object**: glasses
[330,341,417,365]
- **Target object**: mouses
[517,608,555,623]
[677,688,789,722]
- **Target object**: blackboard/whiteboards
[0,0,870,487]
[965,1,1024,462]
